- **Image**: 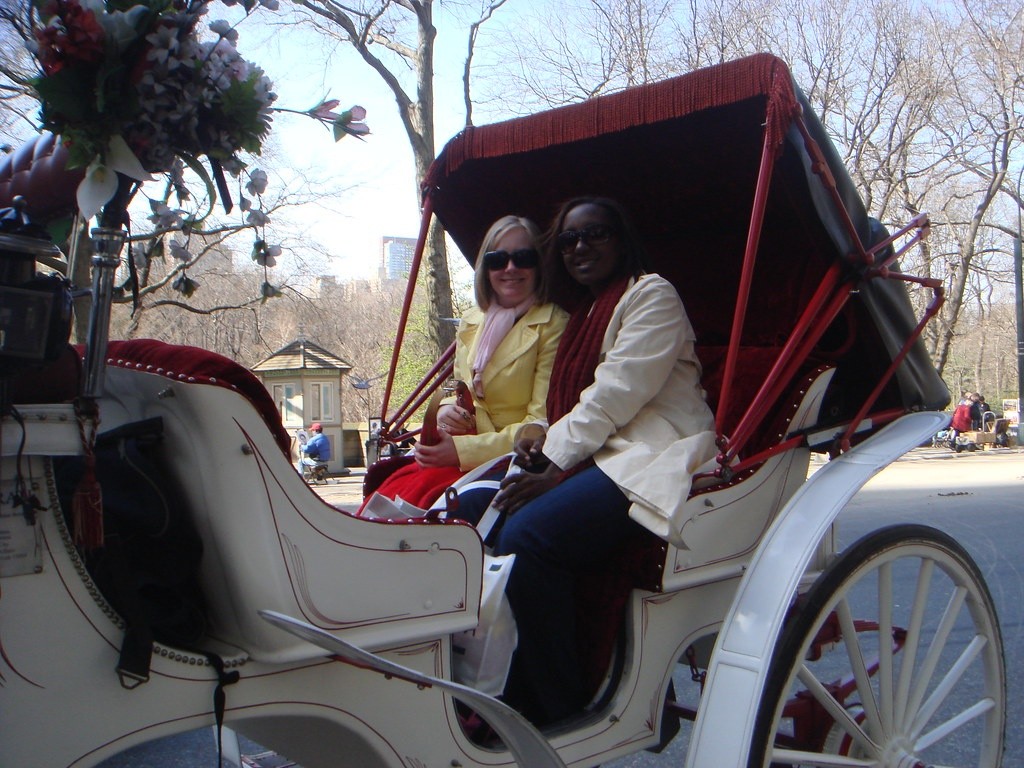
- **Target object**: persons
[356,196,740,733]
[298,423,331,477]
[950,390,991,448]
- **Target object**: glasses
[557,224,613,255]
[484,249,538,271]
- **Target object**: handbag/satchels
[359,452,518,696]
[421,379,477,447]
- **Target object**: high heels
[459,710,494,744]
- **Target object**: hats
[308,423,322,430]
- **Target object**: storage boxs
[959,430,995,442]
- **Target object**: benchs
[62,341,482,663]
[595,343,839,594]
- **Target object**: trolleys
[955,411,997,452]
